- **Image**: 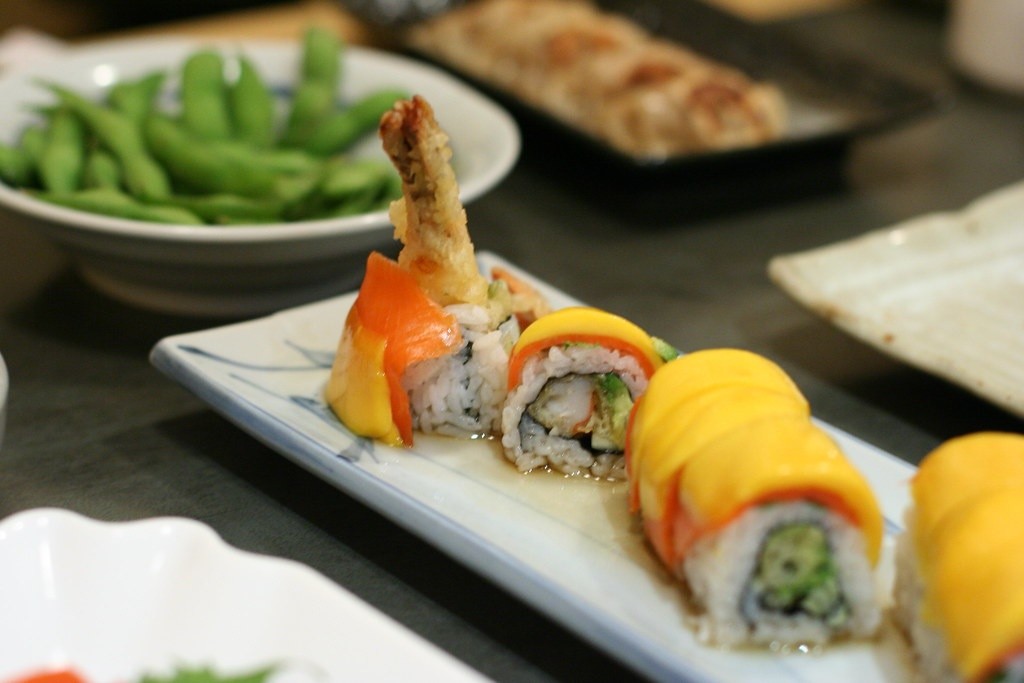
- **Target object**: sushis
[433,0,784,161]
[321,251,1024,683]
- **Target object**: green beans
[0,26,418,225]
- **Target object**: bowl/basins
[0,40,519,312]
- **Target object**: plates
[149,250,1024,683]
[766,183,1024,424]
[3,507,498,682]
[351,1,938,220]
